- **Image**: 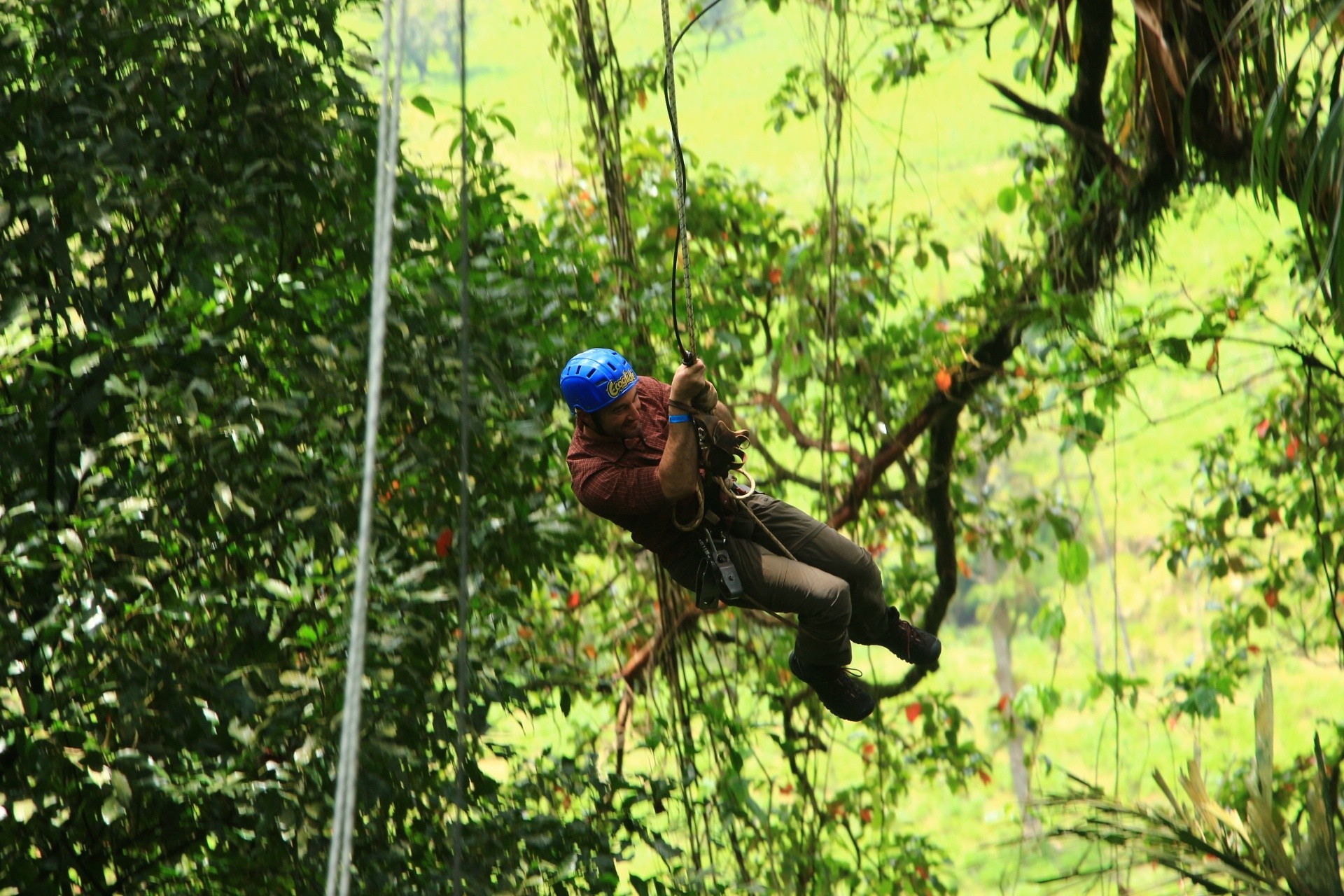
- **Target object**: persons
[560,347,942,722]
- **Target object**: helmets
[560,348,639,415]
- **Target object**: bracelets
[669,414,692,423]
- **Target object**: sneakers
[849,606,941,665]
[790,650,874,721]
